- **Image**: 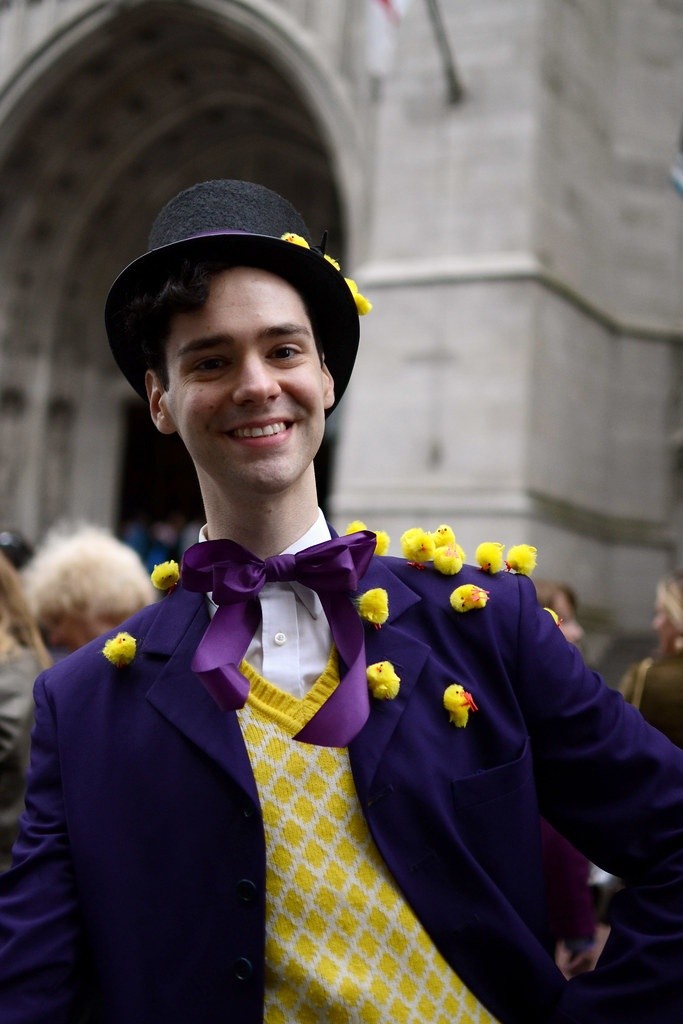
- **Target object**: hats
[104,182,371,417]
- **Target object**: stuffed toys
[103,631,137,666]
[443,683,477,728]
[475,541,537,575]
[401,524,465,575]
[449,584,489,612]
[357,588,389,628]
[365,661,400,699]
[149,559,181,591]
[346,520,389,556]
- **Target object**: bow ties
[178,530,378,746]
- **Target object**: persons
[0,549,52,871]
[17,533,156,653]
[616,569,683,747]
[0,178,683,1023]
[533,577,597,976]
